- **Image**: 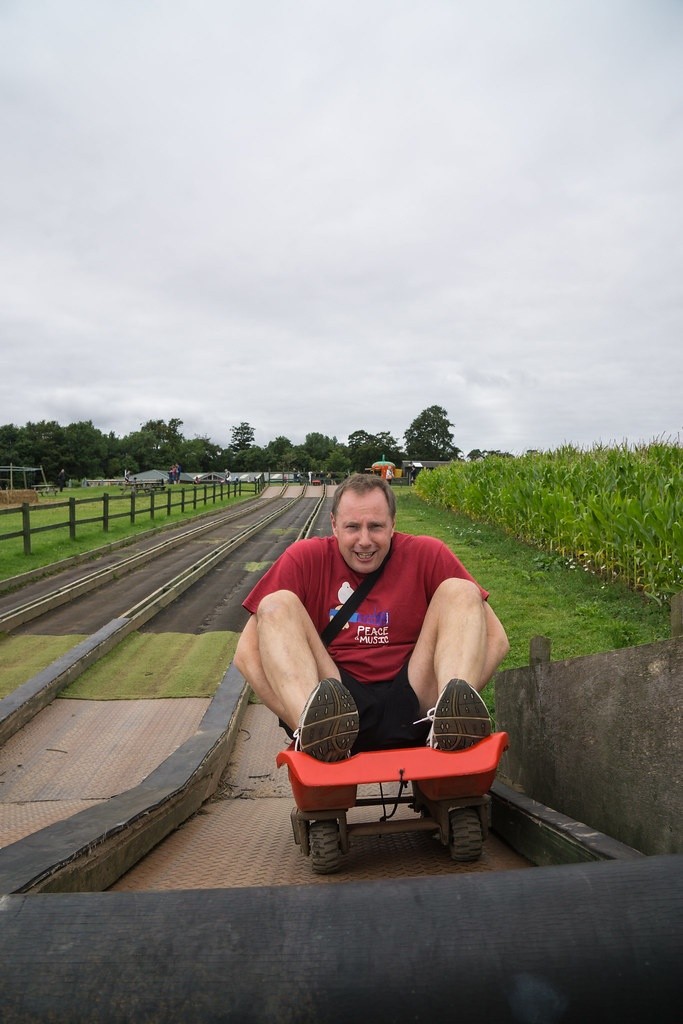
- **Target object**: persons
[125,471,130,483]
[176,463,181,484]
[325,473,331,485]
[170,465,177,484]
[58,469,65,492]
[225,469,231,483]
[195,477,199,484]
[233,474,511,762]
[386,466,394,485]
[330,473,336,485]
[411,466,418,484]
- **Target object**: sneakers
[293,678,360,763]
[412,677,491,751]
